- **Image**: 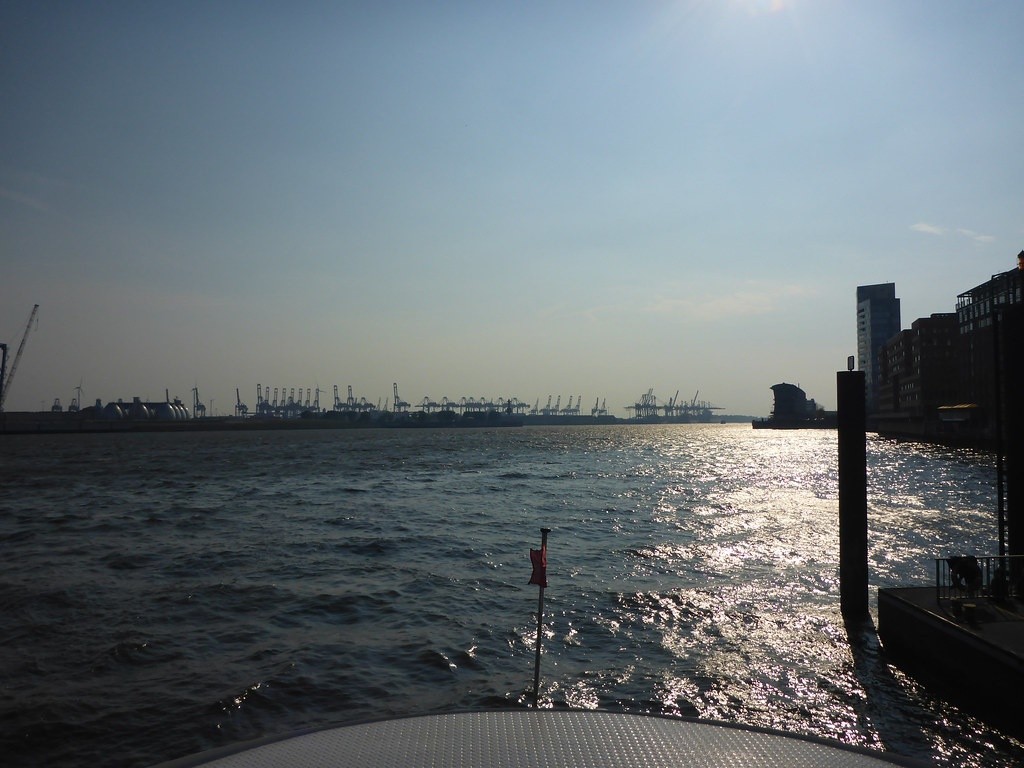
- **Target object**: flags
[527,547,549,588]
